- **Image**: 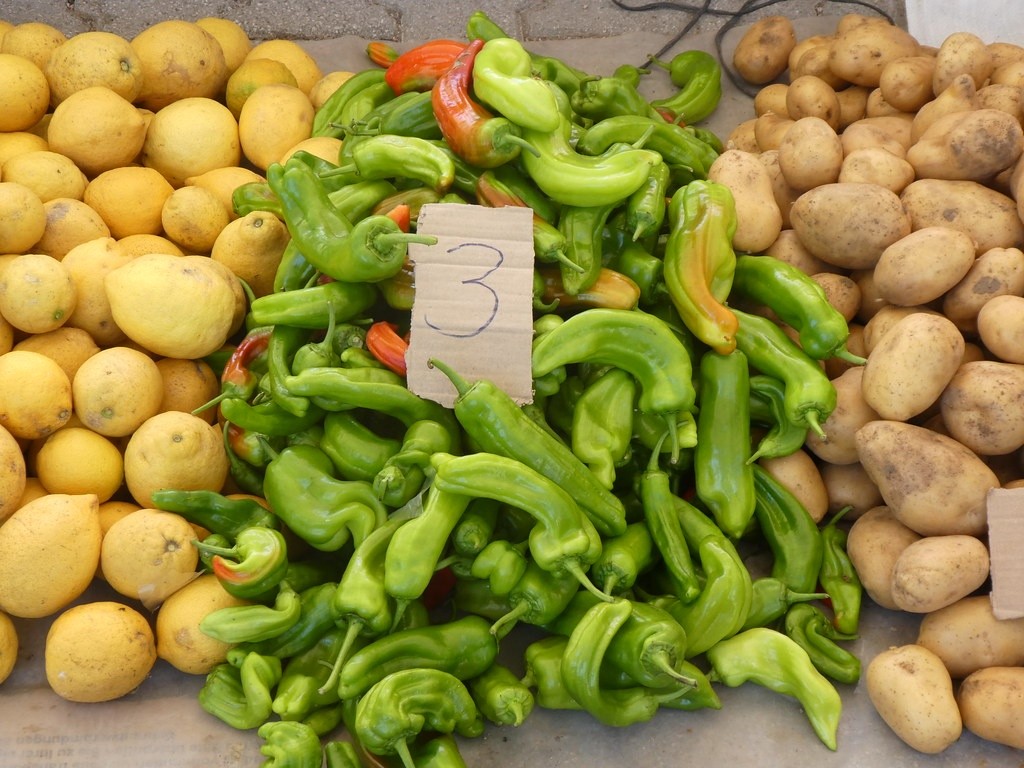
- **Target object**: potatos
[703,9,1024,756]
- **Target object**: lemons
[0,15,360,704]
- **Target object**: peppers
[149,9,864,768]
